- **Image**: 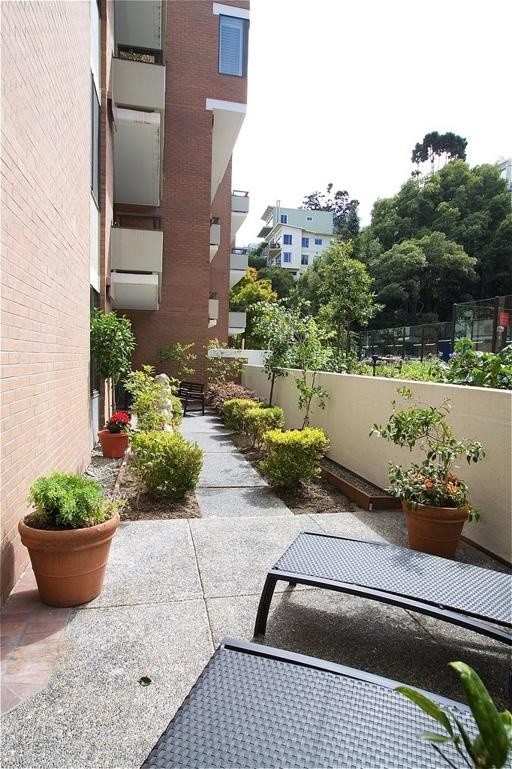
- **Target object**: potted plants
[18,468,120,611]
[366,384,487,561]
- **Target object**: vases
[96,429,130,459]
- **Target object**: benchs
[252,528,512,646]
[174,380,206,416]
[138,634,512,768]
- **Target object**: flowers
[105,410,130,434]
